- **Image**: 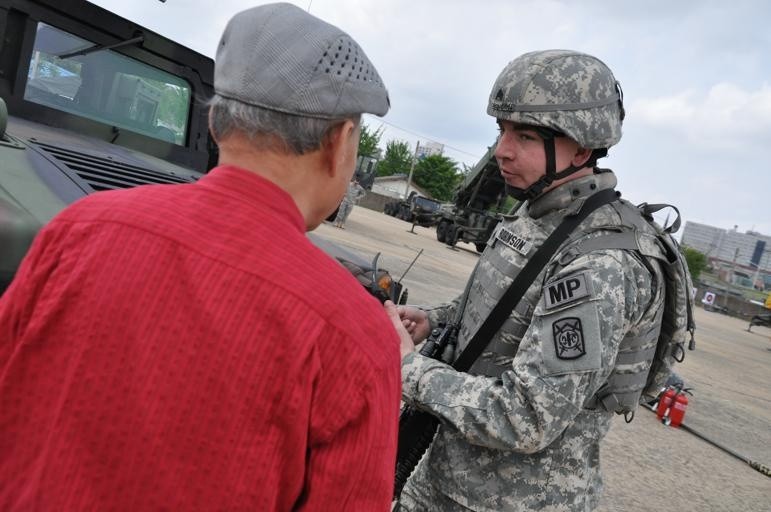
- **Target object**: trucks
[384,134,510,252]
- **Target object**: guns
[392,322,459,503]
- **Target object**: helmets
[486,48,625,148]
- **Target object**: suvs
[1,2,396,303]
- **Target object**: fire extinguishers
[656,383,694,428]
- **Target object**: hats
[211,2,391,122]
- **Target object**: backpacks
[540,203,696,406]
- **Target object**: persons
[333,178,365,228]
[0,1,402,512]
[382,49,668,510]
